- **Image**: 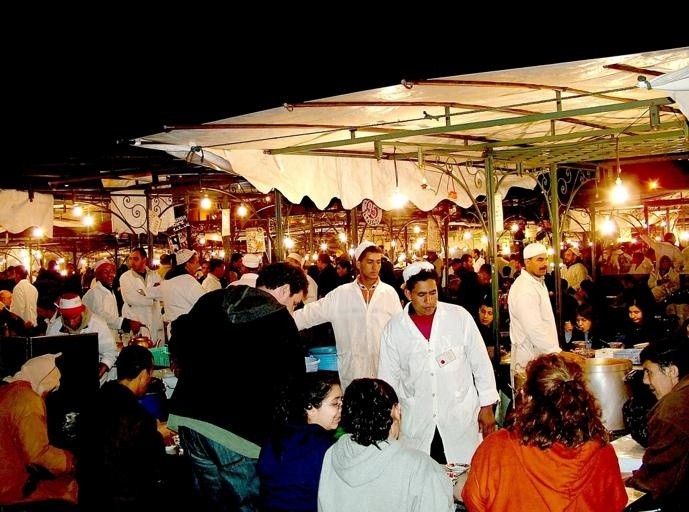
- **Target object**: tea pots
[128,324,161,349]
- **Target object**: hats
[403,261,434,284]
[569,247,582,257]
[94,259,112,272]
[355,241,379,262]
[242,252,259,269]
[523,243,547,260]
[176,249,195,266]
[59,293,86,317]
[287,253,304,265]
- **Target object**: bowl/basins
[572,340,589,350]
[607,341,623,348]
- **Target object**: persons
[256,371,343,512]
[317,379,455,512]
[460,353,629,511]
[0,240,689,413]
[169,262,308,512]
[375,261,501,466]
[80,345,167,511]
[0,352,80,512]
[626,335,689,512]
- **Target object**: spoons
[571,322,584,331]
